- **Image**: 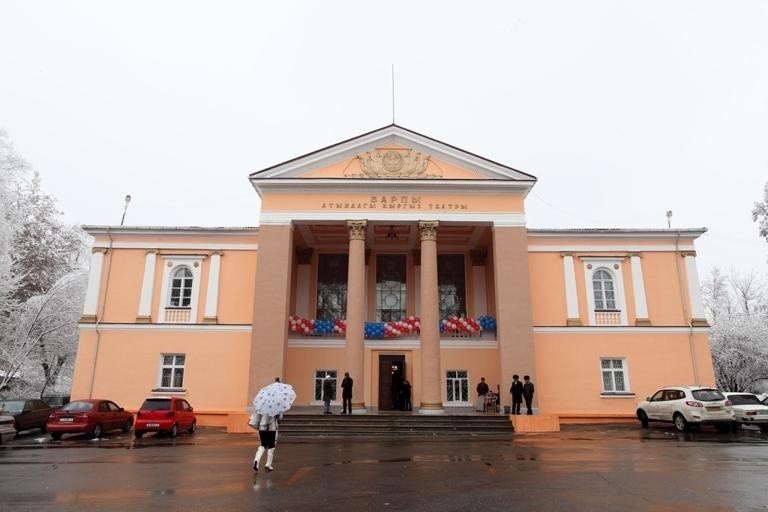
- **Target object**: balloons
[438,314,496,334]
[286,312,348,338]
[361,315,422,339]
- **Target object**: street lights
[121,195,131,226]
[666,210,672,228]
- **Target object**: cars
[0,397,134,440]
[135,396,197,438]
[636,386,768,433]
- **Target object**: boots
[265,448,276,471]
[253,445,265,472]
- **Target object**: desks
[484,397,496,414]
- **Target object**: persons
[474,377,488,413]
[485,384,499,412]
[251,411,283,473]
[340,371,353,415]
[322,374,333,415]
[273,376,281,384]
[398,381,412,411]
[522,375,534,414]
[396,380,411,412]
[509,375,523,415]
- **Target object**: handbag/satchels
[247,413,259,430]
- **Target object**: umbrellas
[250,383,298,418]
